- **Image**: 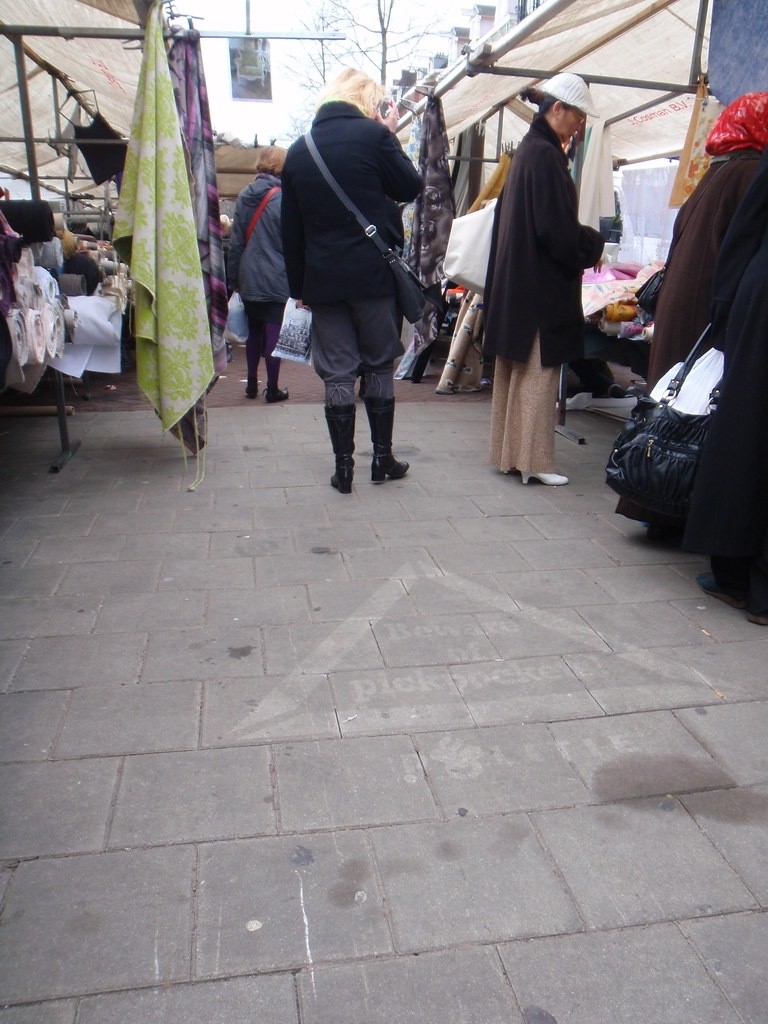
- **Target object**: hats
[542,72,601,118]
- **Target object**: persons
[280,67,424,494]
[615,92,768,626]
[226,145,291,403]
[482,73,605,486]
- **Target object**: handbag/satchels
[606,324,726,517]
[388,253,428,324]
[224,291,249,345]
[270,297,313,366]
[443,198,499,295]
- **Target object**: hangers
[57,78,95,113]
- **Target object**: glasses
[568,108,586,125]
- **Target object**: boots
[325,403,356,493]
[364,396,410,481]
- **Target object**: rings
[397,117,399,120]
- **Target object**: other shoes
[262,377,289,402]
[696,572,748,609]
[745,611,768,625]
[245,377,258,398]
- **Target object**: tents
[0,0,198,235]
[390,0,768,338]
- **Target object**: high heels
[504,468,517,473]
[521,470,568,485]
